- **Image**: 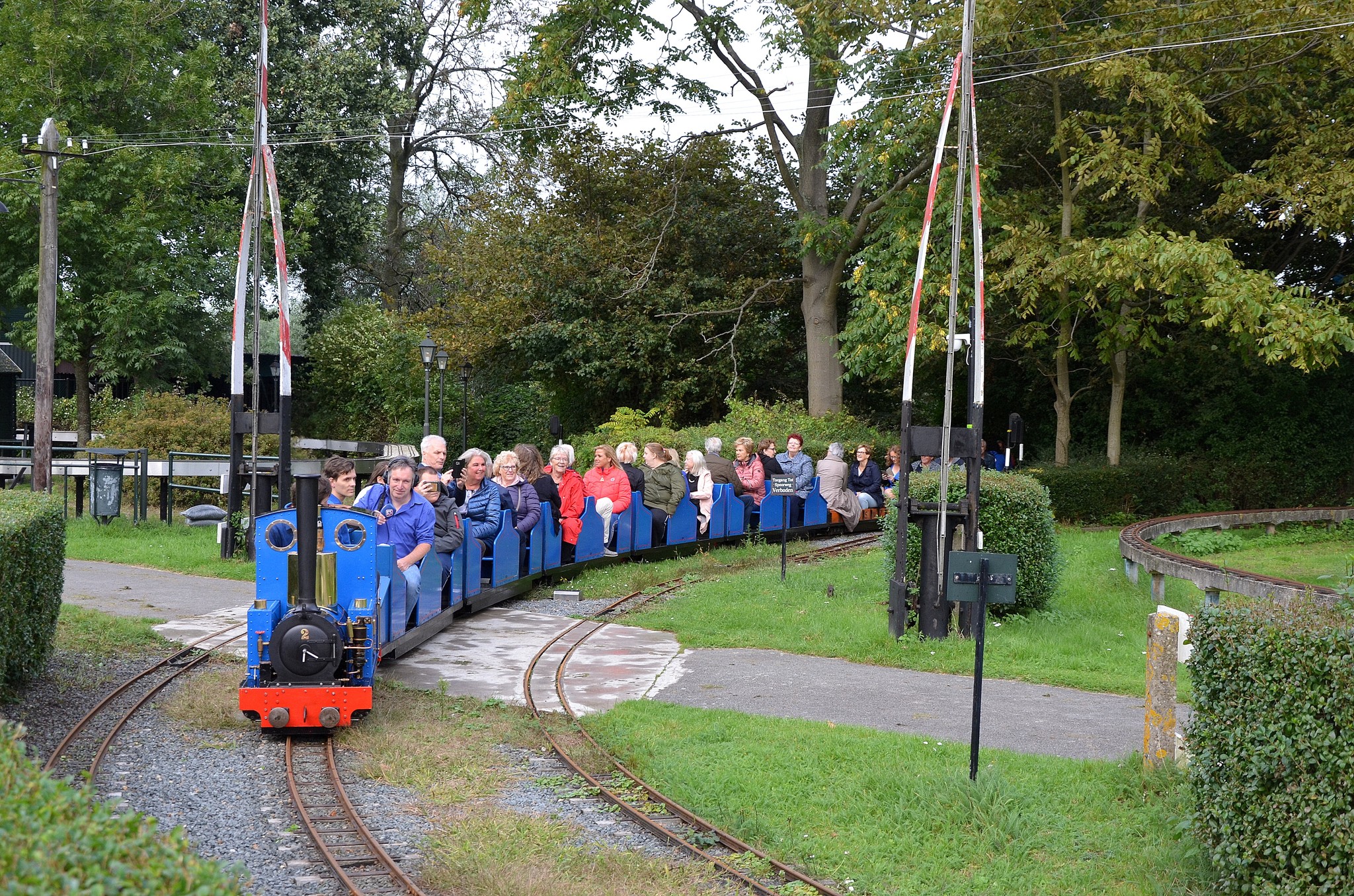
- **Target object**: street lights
[459,356,474,456]
[434,344,449,437]
[418,331,437,435]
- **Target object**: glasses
[552,457,568,462]
[890,456,898,459]
[856,451,868,455]
[499,465,517,470]
[766,447,776,451]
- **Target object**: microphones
[381,503,396,519]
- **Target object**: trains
[240,471,887,729]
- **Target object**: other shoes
[603,546,618,557]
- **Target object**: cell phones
[881,467,894,478]
[424,481,441,492]
[452,458,465,478]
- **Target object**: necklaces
[860,464,866,471]
[688,471,697,482]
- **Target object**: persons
[285,433,1014,650]
